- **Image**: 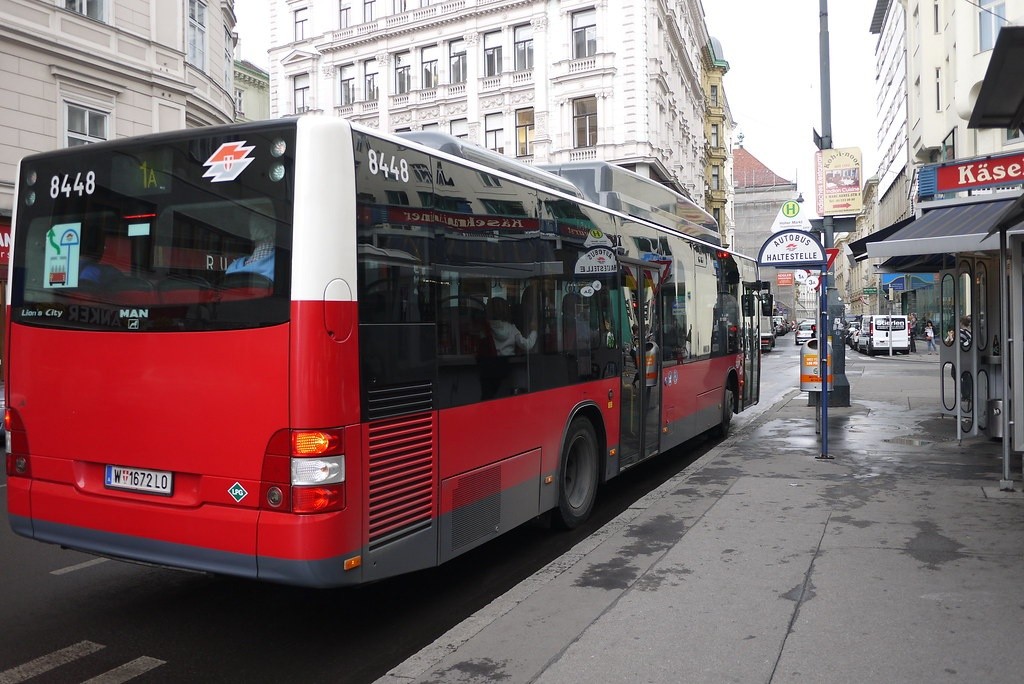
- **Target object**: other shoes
[935,352,939,355]
[928,352,932,355]
[632,382,636,388]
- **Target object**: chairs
[52,257,590,361]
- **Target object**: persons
[485,295,539,356]
[908,312,919,354]
[944,313,973,402]
[56,218,131,288]
[631,324,654,390]
[924,320,941,356]
[604,315,616,375]
[226,205,282,281]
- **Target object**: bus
[0,114,772,588]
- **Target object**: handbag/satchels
[927,336,931,341]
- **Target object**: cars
[845,322,861,350]
[773,316,789,338]
[793,322,816,346]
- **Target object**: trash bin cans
[800,338,834,392]
[986,398,1011,438]
[636,341,659,387]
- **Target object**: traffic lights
[727,325,738,354]
[718,250,731,282]
[644,300,651,320]
[811,325,816,338]
[626,299,633,320]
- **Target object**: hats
[248,207,276,241]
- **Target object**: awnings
[847,214,915,264]
[980,193,1023,241]
[866,198,1022,260]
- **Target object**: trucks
[760,300,776,352]
[857,315,911,356]
